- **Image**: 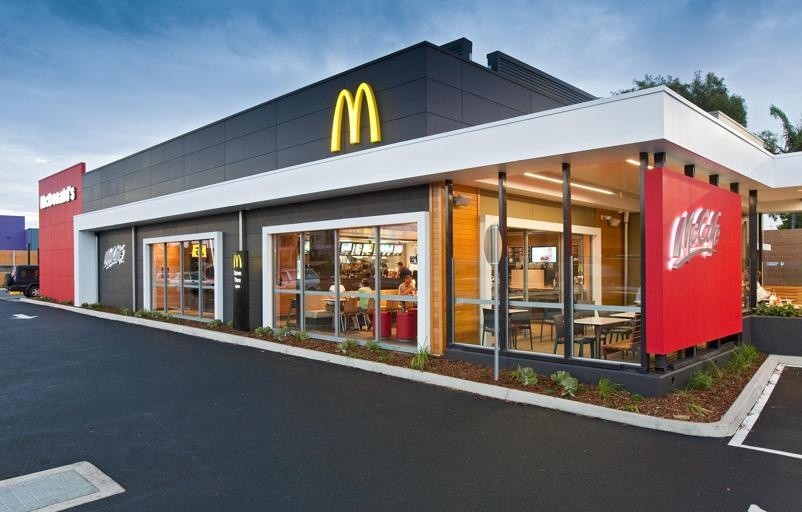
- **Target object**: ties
[530,245,558,263]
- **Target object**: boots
[275,267,321,296]
[1,264,39,297]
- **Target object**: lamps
[481,298,642,360]
[340,294,414,334]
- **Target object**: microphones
[373,308,417,342]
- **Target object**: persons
[355,278,372,330]
[399,274,415,305]
[396,261,411,281]
[325,277,346,329]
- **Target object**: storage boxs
[304,294,332,331]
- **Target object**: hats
[155,263,215,297]
